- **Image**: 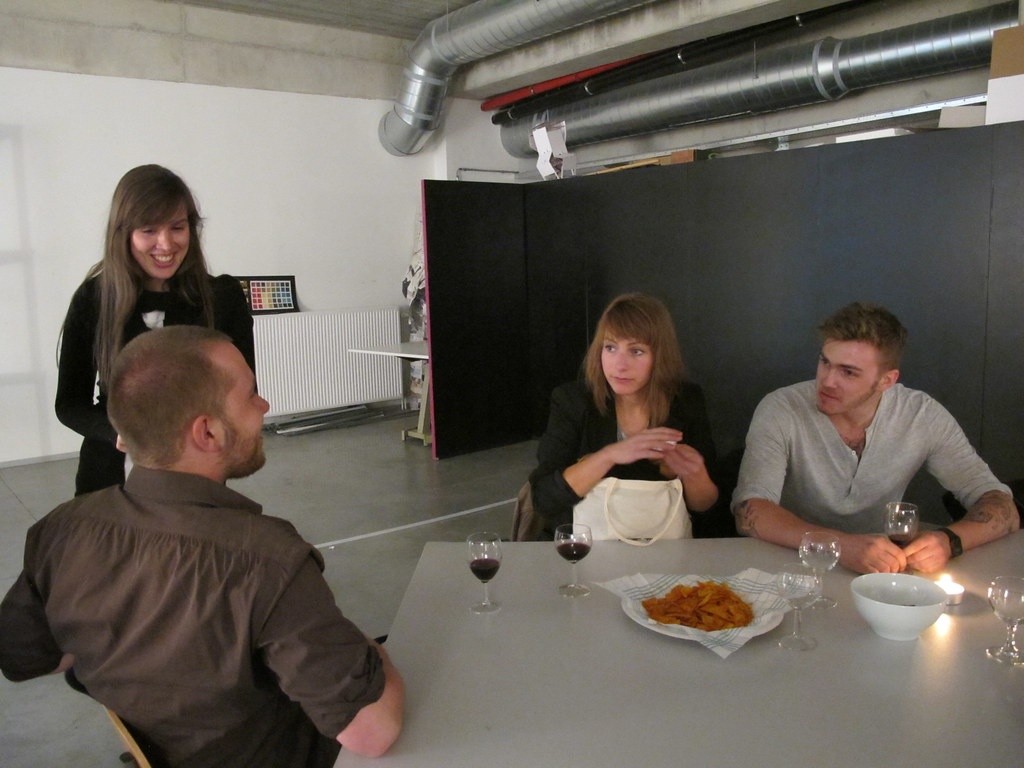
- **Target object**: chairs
[63,667,163,768]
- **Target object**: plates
[621,597,784,640]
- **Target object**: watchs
[938,528,962,556]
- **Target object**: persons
[529,293,722,541]
[53,165,258,498]
[0,325,404,768]
[729,302,1020,575]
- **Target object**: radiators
[252,304,404,420]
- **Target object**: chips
[642,580,755,632]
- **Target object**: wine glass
[884,502,918,550]
[466,532,503,614]
[554,524,593,597]
[985,576,1024,665]
[776,563,823,652]
[798,532,841,609]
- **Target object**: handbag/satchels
[572,477,693,547]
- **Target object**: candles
[934,572,966,608]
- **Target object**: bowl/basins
[851,573,949,642]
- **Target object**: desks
[327,542,1024,768]
[347,341,433,449]
